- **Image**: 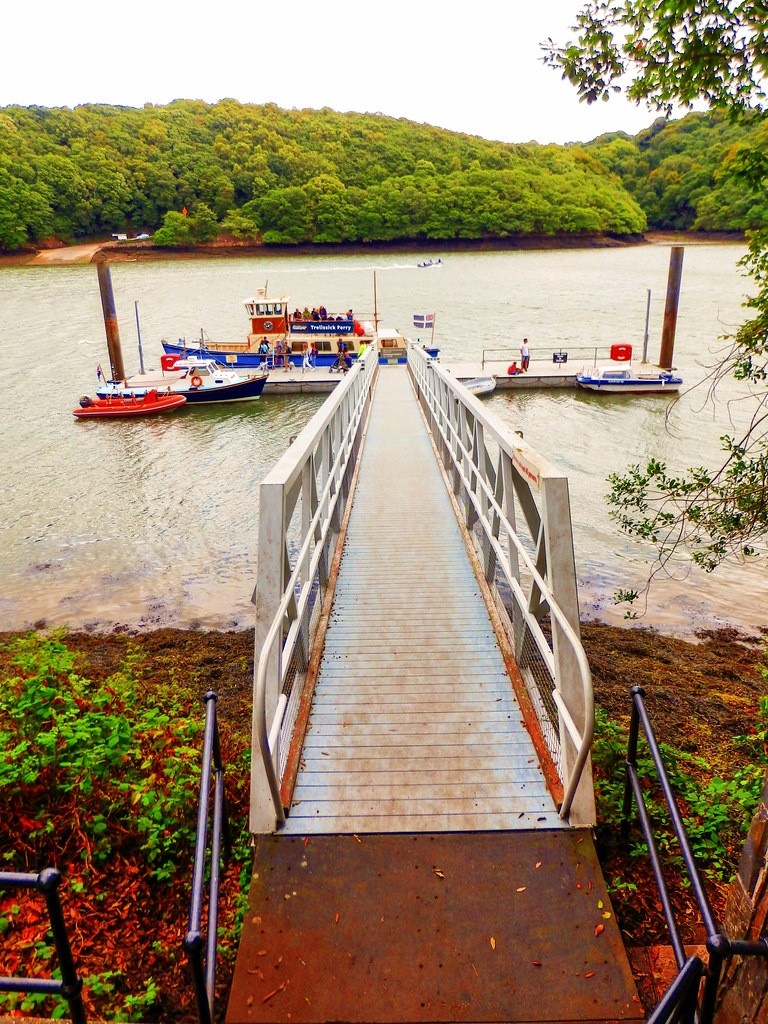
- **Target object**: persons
[293,305,354,321]
[256,336,367,376]
[508,338,529,375]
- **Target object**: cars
[136,234,149,239]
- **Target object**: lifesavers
[190,375,202,387]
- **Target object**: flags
[96,366,102,380]
[413,314,434,328]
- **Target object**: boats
[160,288,440,368]
[576,364,682,393]
[416,259,441,268]
[95,356,269,404]
[453,374,496,402]
[71,386,187,417]
